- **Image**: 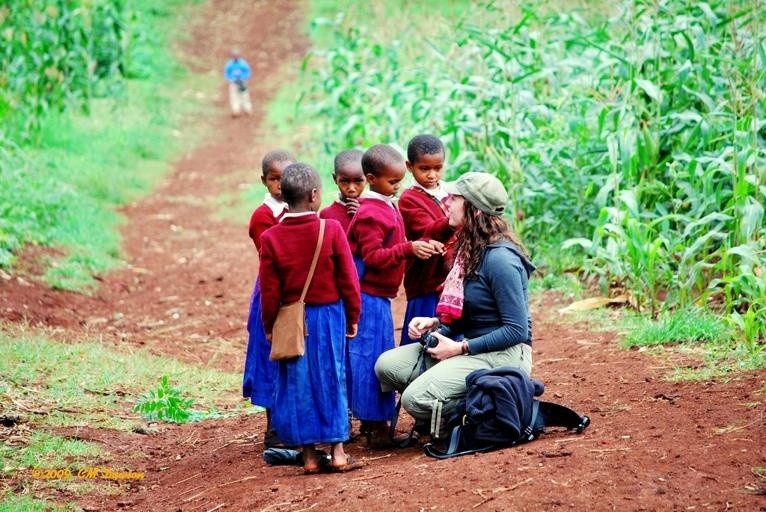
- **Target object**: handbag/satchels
[268,300,306,361]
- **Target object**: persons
[373,170,539,444]
[317,148,370,275]
[240,150,298,449]
[339,142,421,451]
[223,48,255,119]
[392,133,467,346]
[257,160,370,476]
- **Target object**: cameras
[417,323,450,350]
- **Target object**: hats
[439,172,508,217]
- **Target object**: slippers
[302,454,351,473]
